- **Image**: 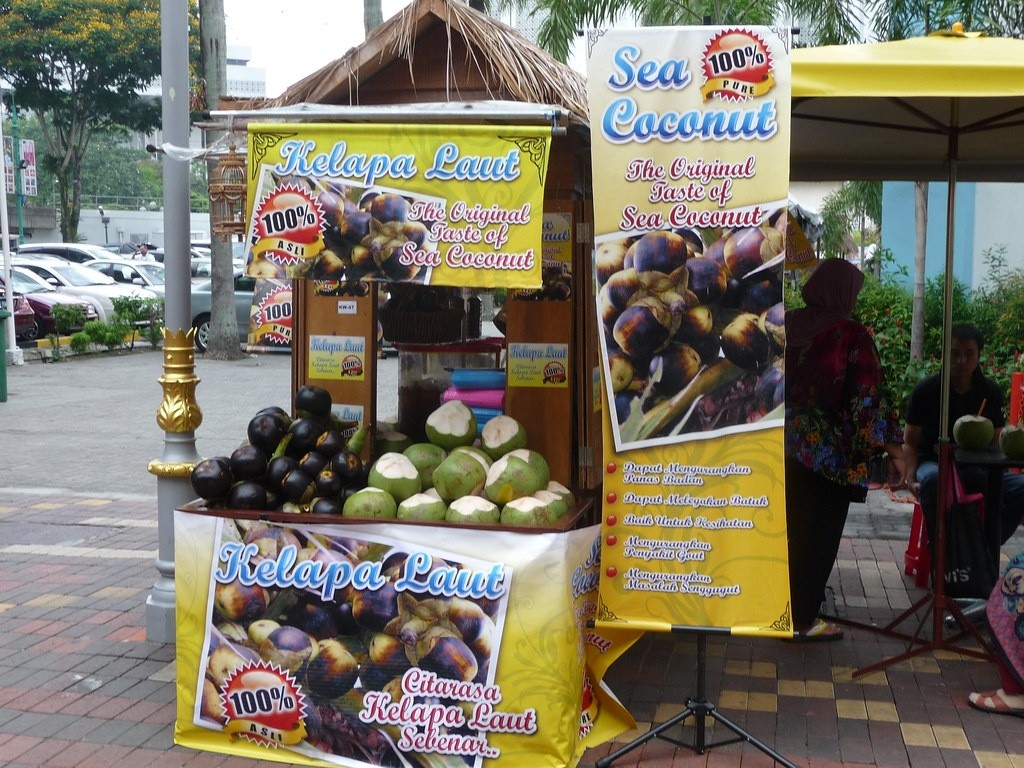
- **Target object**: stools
[903,494,933,589]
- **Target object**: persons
[134,245,156,262]
[901,320,1007,628]
[783,259,910,643]
[967,553,1024,715]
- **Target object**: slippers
[783,618,844,641]
[970,687,1024,717]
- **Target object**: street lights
[13,160,30,242]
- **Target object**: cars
[81,259,196,299]
[16,244,128,263]
[190,271,255,354]
[0,277,34,337]
[0,253,159,329]
[0,265,99,340]
[105,241,245,290]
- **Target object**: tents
[792,22,1023,646]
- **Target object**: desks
[947,441,1024,648]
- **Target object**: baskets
[377,288,463,342]
